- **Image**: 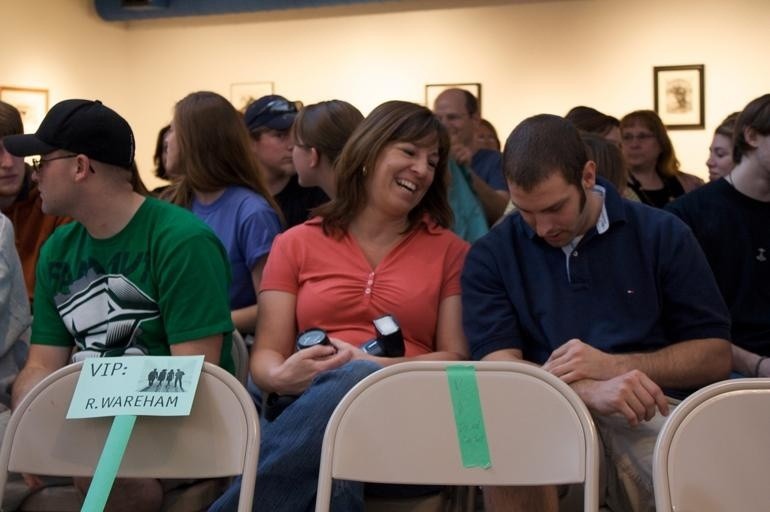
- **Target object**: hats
[565,107,620,129]
[3,99,135,167]
[245,96,298,131]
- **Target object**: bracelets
[754,355,770,378]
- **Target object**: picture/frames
[423,79,483,118]
[1,84,50,135]
[651,62,708,132]
[228,78,275,114]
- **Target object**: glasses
[433,111,467,122]
[621,133,654,142]
[250,101,296,128]
[32,155,96,174]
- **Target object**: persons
[660,93,770,399]
[461,114,733,512]
[207,99,472,512]
[0,99,235,512]
[1,87,769,424]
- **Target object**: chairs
[651,375,770,511]
[0,356,265,512]
[313,359,607,512]
